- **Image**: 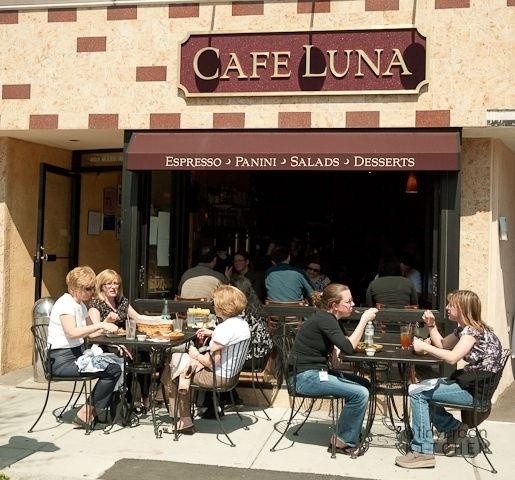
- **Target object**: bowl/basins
[136,335,146,342]
[365,348,376,356]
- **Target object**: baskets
[138,324,173,337]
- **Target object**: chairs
[265,299,304,335]
[230,320,286,420]
[375,303,418,333]
[28,296,111,436]
[270,335,345,458]
[174,296,207,320]
[406,349,511,475]
[170,334,257,446]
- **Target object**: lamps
[404,171,419,194]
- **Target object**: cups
[126,320,136,340]
[173,319,183,331]
[400,326,411,349]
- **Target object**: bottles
[161,299,170,319]
[365,321,373,344]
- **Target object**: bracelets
[427,325,434,328]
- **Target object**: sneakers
[394,450,436,469]
[446,422,469,457]
[72,412,108,430]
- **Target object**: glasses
[102,282,121,288]
[334,296,353,304]
[85,285,95,291]
[306,267,321,272]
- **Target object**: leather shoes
[197,405,222,419]
[163,423,198,435]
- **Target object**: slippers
[327,443,365,456]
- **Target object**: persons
[204,274,274,404]
[176,242,423,309]
[395,290,503,469]
[45,265,122,430]
[154,284,251,435]
[88,269,152,413]
[285,283,378,456]
